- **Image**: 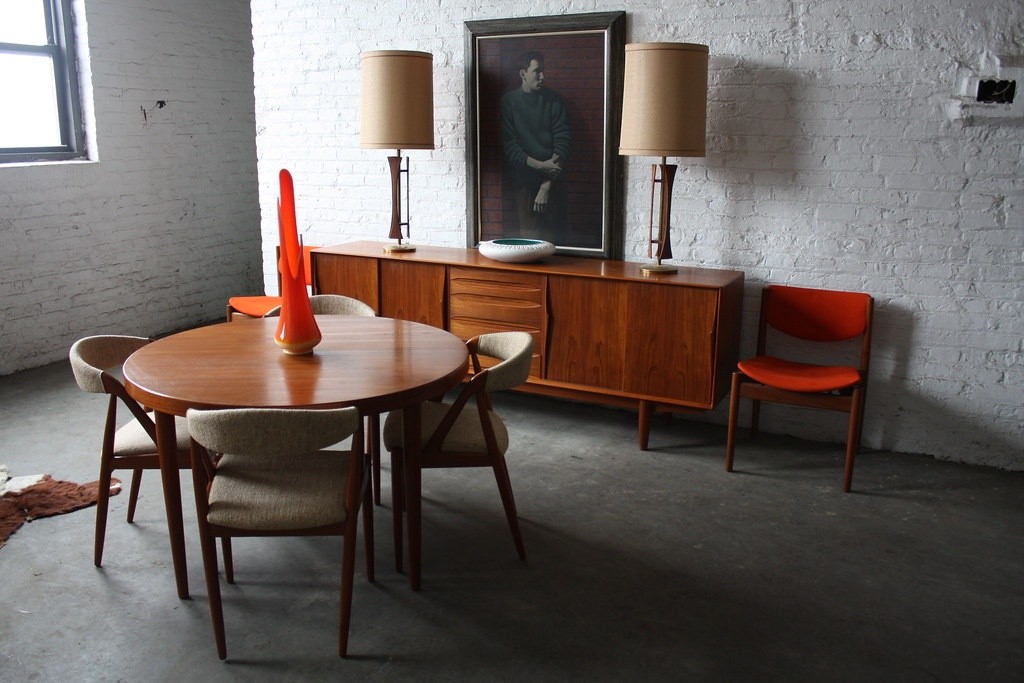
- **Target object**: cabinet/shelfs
[309,240,745,449]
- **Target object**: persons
[497,51,578,243]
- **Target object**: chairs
[383,332,536,572]
[262,294,380,505]
[187,407,375,660]
[227,244,318,319]
[726,285,875,492]
[70,334,220,578]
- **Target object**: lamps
[618,43,709,275]
[358,51,434,253]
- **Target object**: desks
[122,314,469,600]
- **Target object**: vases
[274,169,322,357]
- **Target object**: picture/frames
[464,10,627,262]
